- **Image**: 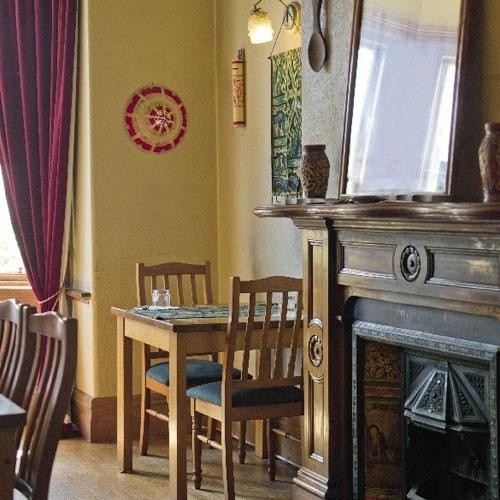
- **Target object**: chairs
[0,298,28,405]
[13,310,77,500]
[186,276,303,500]
[136,261,254,463]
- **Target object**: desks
[0,392,28,500]
[111,301,303,498]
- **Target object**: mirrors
[337,0,472,204]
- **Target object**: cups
[151,289,170,310]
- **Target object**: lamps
[247,0,301,45]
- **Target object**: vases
[298,144,330,204]
[479,123,500,204]
